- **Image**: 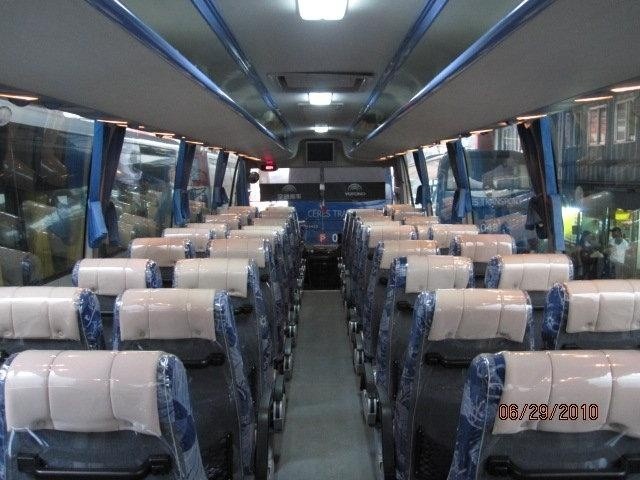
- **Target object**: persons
[579,226,630,281]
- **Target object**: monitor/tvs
[306,141,336,168]
